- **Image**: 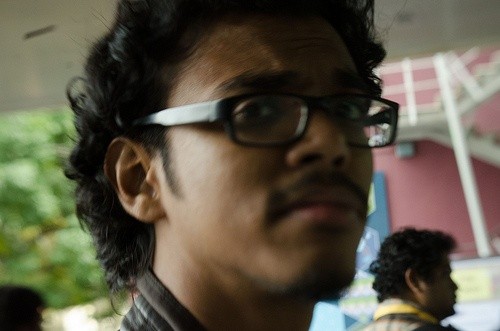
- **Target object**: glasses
[136,90,400,151]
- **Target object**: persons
[365,226,459,331]
[63,1,402,331]
[0,286,46,331]
